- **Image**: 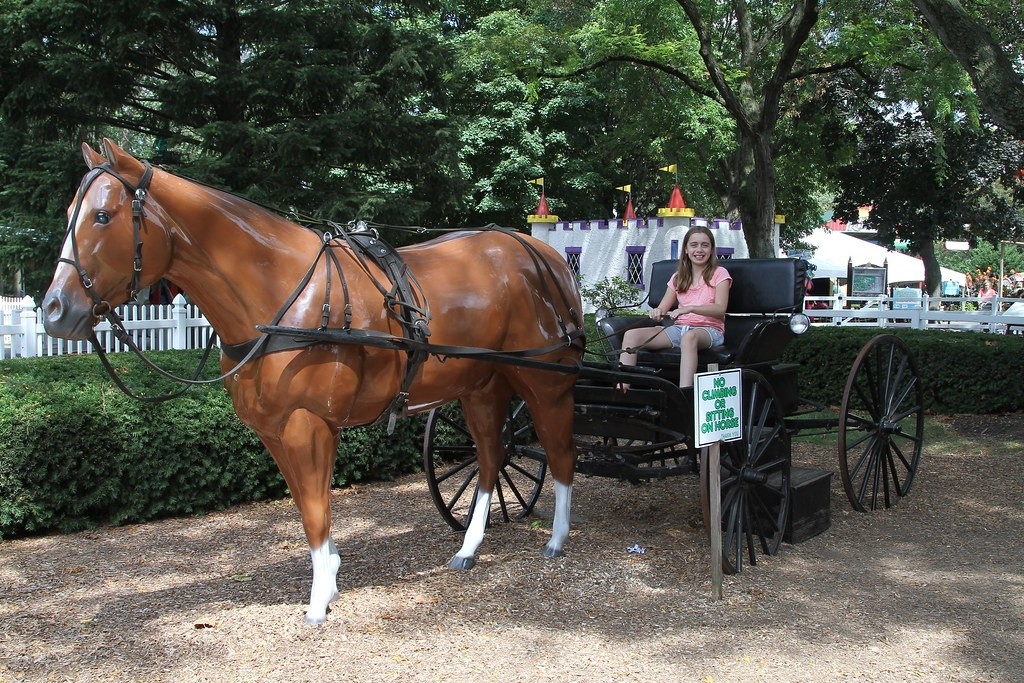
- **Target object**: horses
[42,136,583,621]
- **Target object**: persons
[815,301,828,309]
[617,226,733,394]
[978,280,996,333]
[804,278,815,323]
[1004,289,1024,335]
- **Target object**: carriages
[36,134,924,627]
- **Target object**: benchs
[598,258,809,364]
